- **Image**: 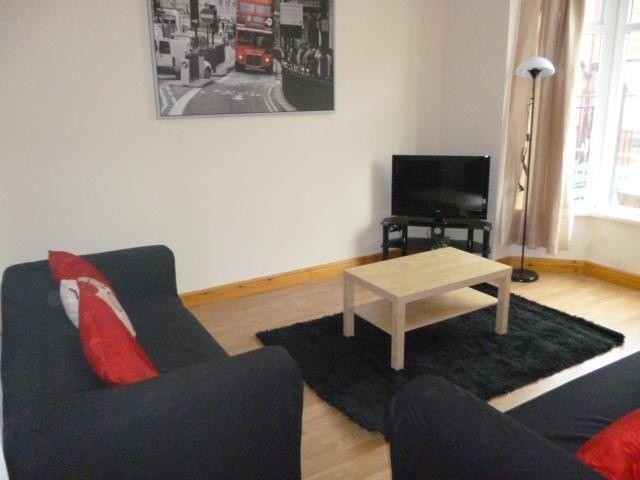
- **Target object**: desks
[381,217,491,258]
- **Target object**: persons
[276,41,333,81]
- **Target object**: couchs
[392,351,640,480]
[0,243,302,480]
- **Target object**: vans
[155,38,185,80]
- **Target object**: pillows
[48,250,116,293]
[59,276,137,337]
[575,408,640,480]
[79,288,160,388]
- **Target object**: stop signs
[199,8,215,25]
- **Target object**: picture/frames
[147,0,337,120]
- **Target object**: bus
[235,1,275,73]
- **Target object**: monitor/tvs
[391,153,491,225]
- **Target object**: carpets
[255,283,624,439]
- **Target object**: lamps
[513,56,556,282]
